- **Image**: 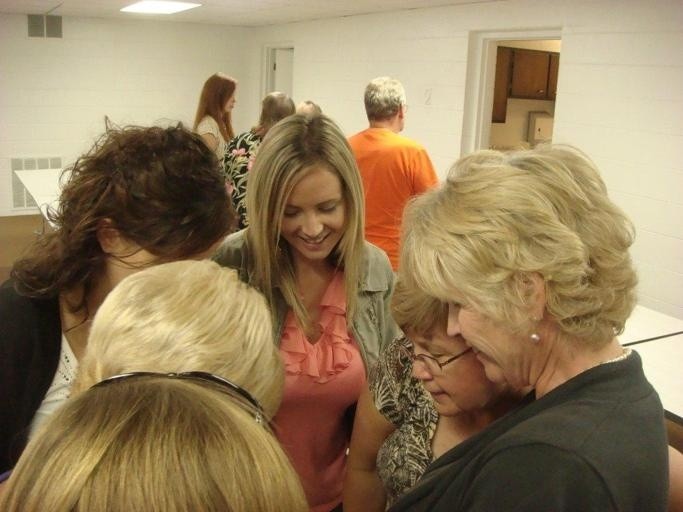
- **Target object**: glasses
[398,342,472,375]
[91,371,266,428]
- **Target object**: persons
[0,374,310,512]
[219,91,295,232]
[347,76,440,271]
[342,276,683,512]
[1,257,311,512]
[192,74,237,162]
[0,121,238,477]
[205,112,396,512]
[391,144,670,511]
[296,102,321,115]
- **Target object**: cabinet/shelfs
[492,46,513,122]
[512,47,559,98]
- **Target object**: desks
[615,305,683,425]
[14,168,74,230]
